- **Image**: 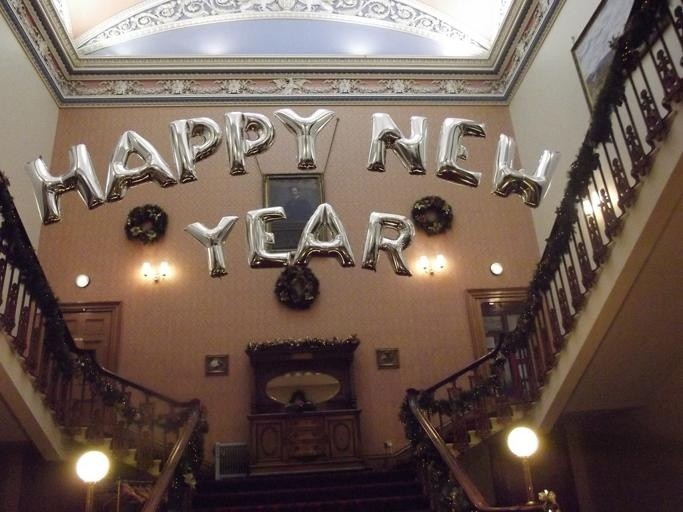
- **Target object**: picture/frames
[569,1,670,118]
[376,347,399,368]
[203,354,229,375]
[263,172,328,255]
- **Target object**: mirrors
[264,371,341,405]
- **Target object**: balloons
[292,202,355,267]
[367,112,429,175]
[223,112,276,176]
[361,211,416,277]
[104,130,178,202]
[273,107,336,169]
[490,132,561,208]
[433,117,487,189]
[244,205,290,268]
[184,216,240,278]
[168,116,224,183]
[24,144,105,225]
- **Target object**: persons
[282,187,313,244]
